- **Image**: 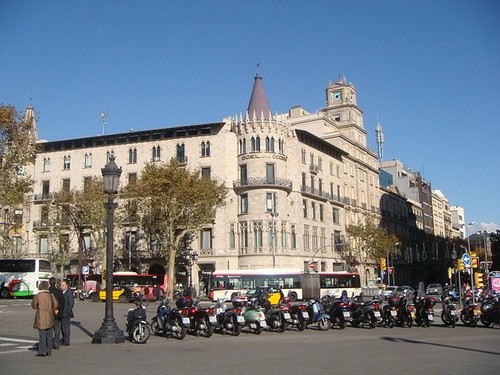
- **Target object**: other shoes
[46,352,51,356]
[63,344,69,346]
[35,353,46,356]
[61,338,64,345]
[53,346,59,349]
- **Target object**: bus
[66,272,176,301]
[199,270,302,301]
[312,271,361,302]
[0,258,53,299]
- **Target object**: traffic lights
[381,258,386,271]
[458,258,464,271]
[475,272,484,289]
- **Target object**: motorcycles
[215,288,500,334]
[151,297,194,340]
[174,292,218,339]
[125,301,152,344]
[192,294,248,336]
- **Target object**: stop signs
[310,263,315,269]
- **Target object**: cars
[381,284,442,299]
[90,286,141,303]
[244,286,284,305]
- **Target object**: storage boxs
[387,297,400,306]
[176,296,193,310]
[425,297,436,307]
[231,296,247,307]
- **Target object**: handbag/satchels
[49,293,59,316]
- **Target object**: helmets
[308,299,315,305]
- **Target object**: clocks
[333,91,341,103]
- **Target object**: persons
[451,284,458,291]
[31,281,58,356]
[48,277,74,349]
[443,283,450,290]
[187,283,193,296]
[144,285,164,306]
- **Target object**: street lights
[92,155,125,344]
[478,230,488,272]
[467,222,476,304]
[388,242,399,286]
